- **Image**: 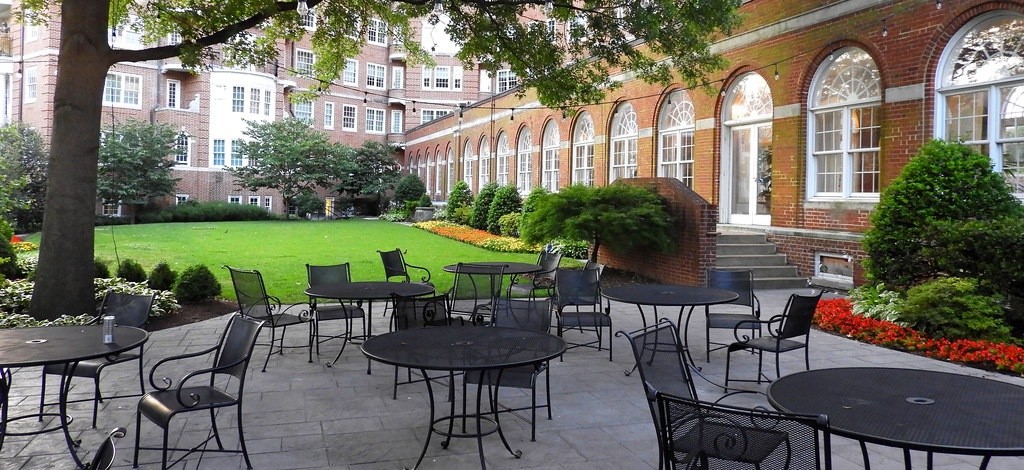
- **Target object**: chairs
[0,246,832,470]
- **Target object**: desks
[360,326,568,470]
[442,261,543,326]
[766,367,1024,470]
[601,284,739,376]
[0,325,150,470]
[304,281,435,374]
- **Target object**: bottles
[103,315,115,344]
